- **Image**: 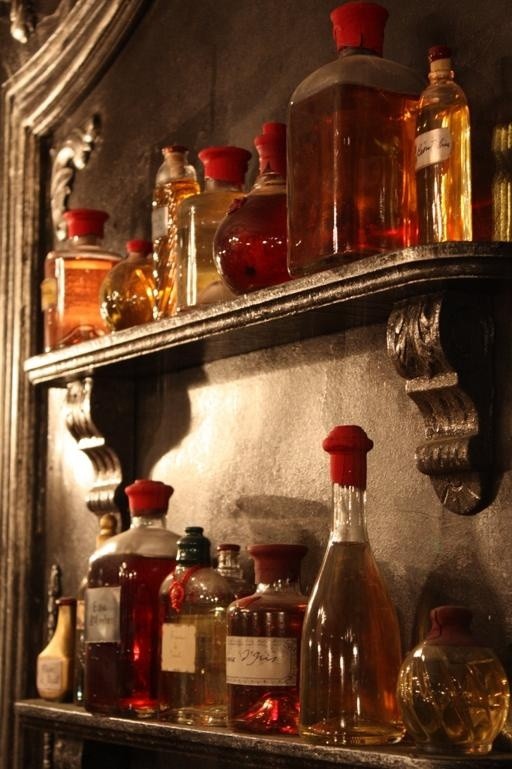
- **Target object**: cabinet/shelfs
[15,241,512,769]
